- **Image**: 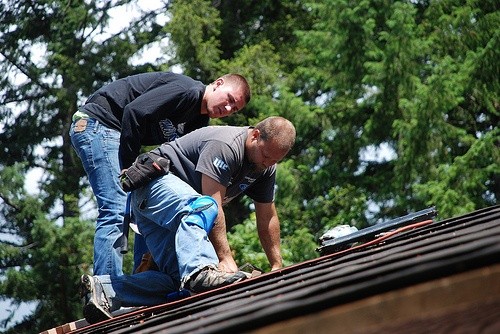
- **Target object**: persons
[79,116,298,325]
[67,70,252,279]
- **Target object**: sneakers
[77,274,113,325]
[185,269,247,294]
